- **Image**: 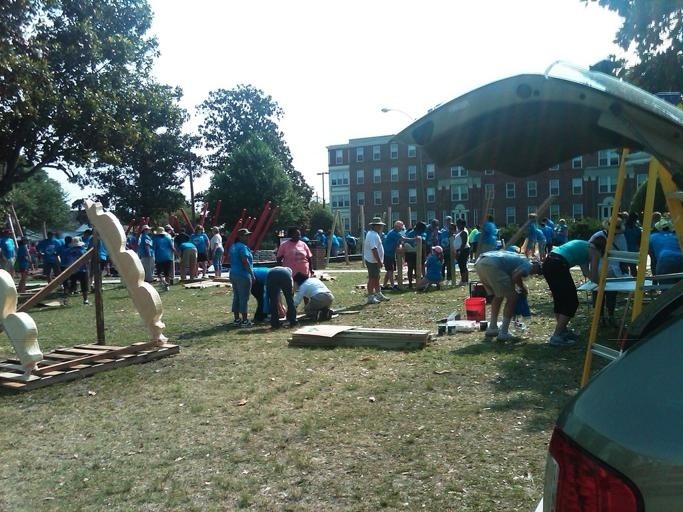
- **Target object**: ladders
[580,140,683,390]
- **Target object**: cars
[531,280,681,512]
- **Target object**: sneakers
[485,327,500,336]
[368,295,380,304]
[233,319,242,327]
[376,292,390,302]
[317,310,322,321]
[497,331,523,342]
[326,310,333,320]
[550,330,577,346]
[422,283,431,292]
[381,285,402,292]
[241,320,255,328]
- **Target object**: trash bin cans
[308,240,326,269]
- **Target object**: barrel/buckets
[464,295,486,322]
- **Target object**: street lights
[187,135,202,229]
[317,171,329,207]
[380,106,414,126]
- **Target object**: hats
[396,221,406,230]
[602,216,625,233]
[237,229,253,236]
[369,217,386,224]
[141,225,173,235]
[68,237,85,247]
[655,220,674,233]
[432,246,444,254]
[211,227,219,232]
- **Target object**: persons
[1,218,225,305]
[229,227,361,328]
[365,209,682,347]
